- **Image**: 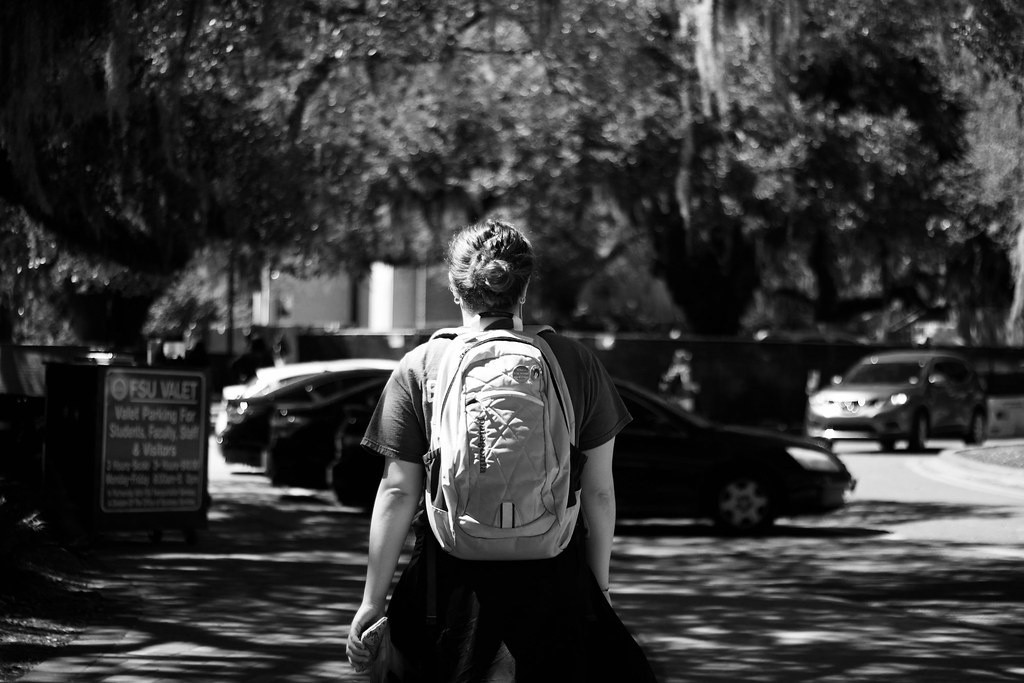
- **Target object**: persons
[661,347,701,413]
[344,217,661,683]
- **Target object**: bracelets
[600,585,611,593]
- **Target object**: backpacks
[423,325,587,559]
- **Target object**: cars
[330,378,857,533]
[213,358,402,468]
[805,350,990,452]
[266,377,394,491]
